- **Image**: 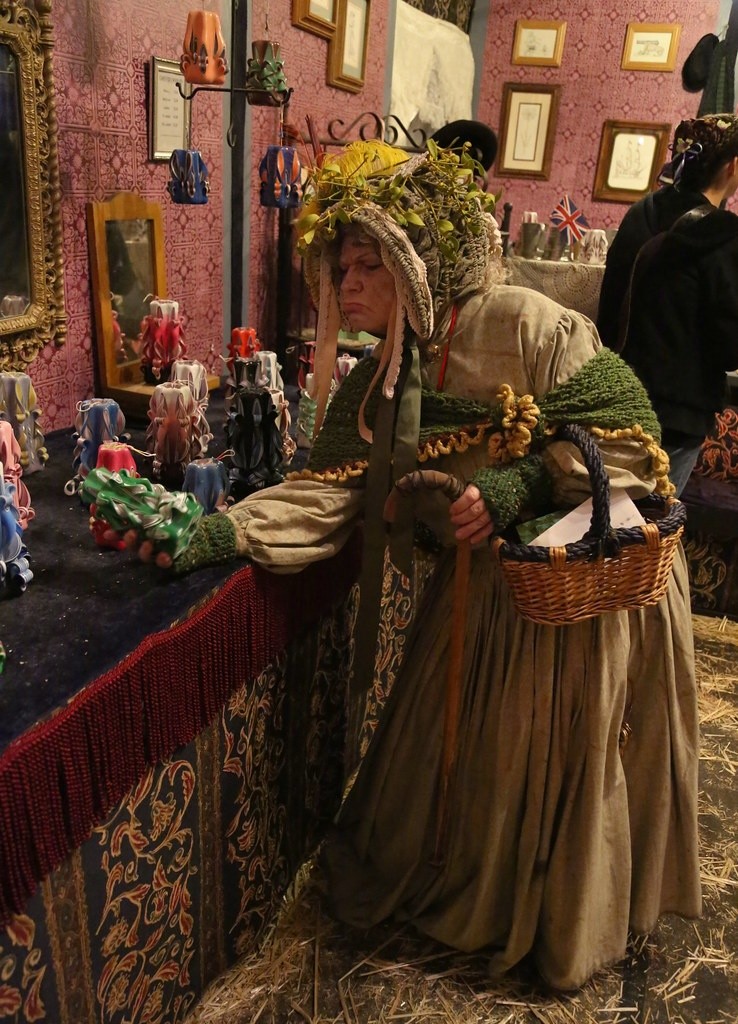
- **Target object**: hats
[295,138,503,444]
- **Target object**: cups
[519,222,548,261]
[546,226,609,266]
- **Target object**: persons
[89,140,703,989]
[596,112,738,501]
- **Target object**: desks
[499,250,609,327]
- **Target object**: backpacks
[614,193,716,411]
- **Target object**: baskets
[490,423,687,627]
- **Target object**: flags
[550,195,590,247]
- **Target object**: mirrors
[0,0,69,373]
[86,192,167,387]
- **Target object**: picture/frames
[621,22,683,72]
[291,0,340,40]
[511,19,568,66]
[494,81,562,182]
[149,55,192,161]
[592,119,673,205]
[326,0,371,95]
